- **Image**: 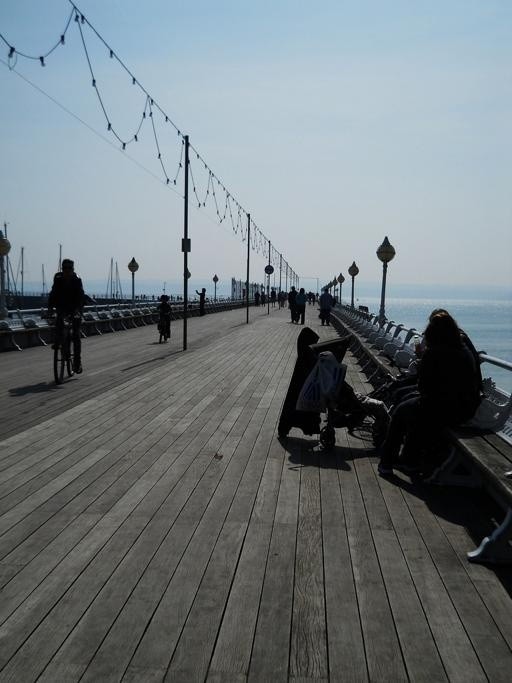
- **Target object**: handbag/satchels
[471,391,512,428]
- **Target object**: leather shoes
[465,537,509,563]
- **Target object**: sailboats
[0,222,132,311]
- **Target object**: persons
[370,306,482,475]
[47,259,87,373]
[288,286,299,323]
[156,294,174,338]
[295,287,307,325]
[254,289,320,308]
[196,287,206,315]
[318,288,333,325]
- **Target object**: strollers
[307,334,390,448]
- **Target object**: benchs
[0,295,233,352]
[330,308,512,562]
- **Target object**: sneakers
[378,461,394,475]
[73,357,80,371]
[394,462,424,473]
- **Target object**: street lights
[0,230,11,312]
[321,285,328,294]
[254,283,258,293]
[376,236,396,321]
[128,257,139,304]
[213,274,218,298]
[183,269,191,302]
[328,281,333,294]
[337,273,345,304]
[348,261,359,307]
[233,277,236,299]
[332,276,338,300]
